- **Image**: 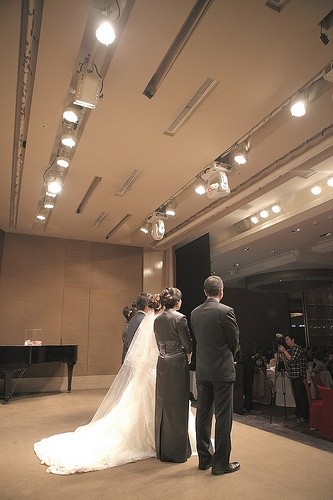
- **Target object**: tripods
[270,352,287,424]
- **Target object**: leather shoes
[211,461,241,476]
[199,461,214,470]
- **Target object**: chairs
[302,371,333,441]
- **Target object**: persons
[189,276,241,475]
[153,287,192,463]
[235,332,333,423]
[32,293,216,476]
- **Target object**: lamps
[140,203,177,240]
[37,64,101,221]
[193,141,249,198]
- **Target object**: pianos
[0,344,80,404]
